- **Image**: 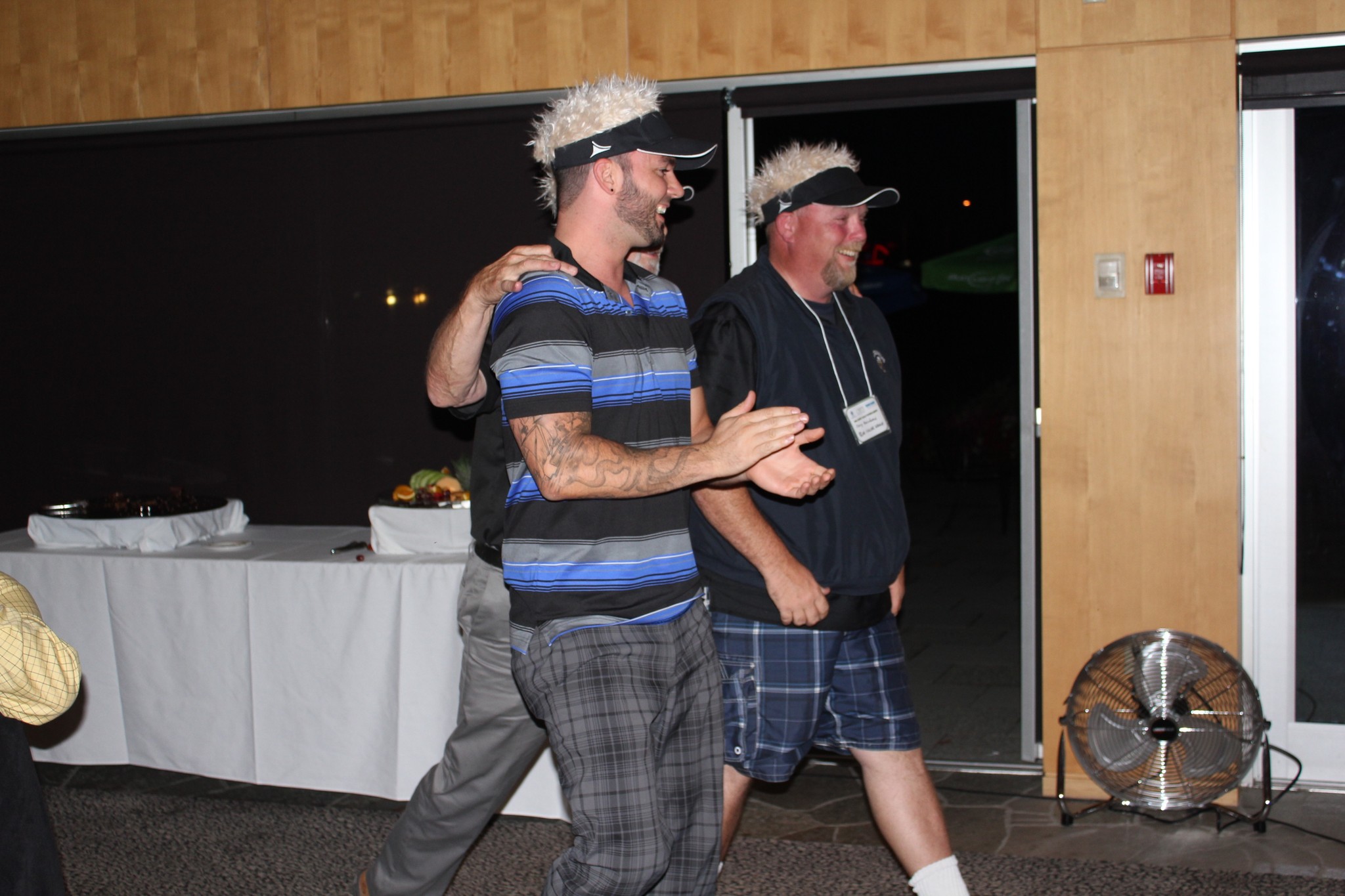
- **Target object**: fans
[1055,629,1272,833]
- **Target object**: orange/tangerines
[392,485,415,501]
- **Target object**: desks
[0,526,575,824]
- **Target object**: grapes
[356,555,364,561]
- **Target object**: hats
[761,167,901,224]
[678,179,694,202]
[551,111,722,171]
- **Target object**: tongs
[330,540,367,555]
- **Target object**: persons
[687,147,971,896]
[0,570,82,726]
[351,77,834,896]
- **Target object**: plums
[396,490,451,508]
